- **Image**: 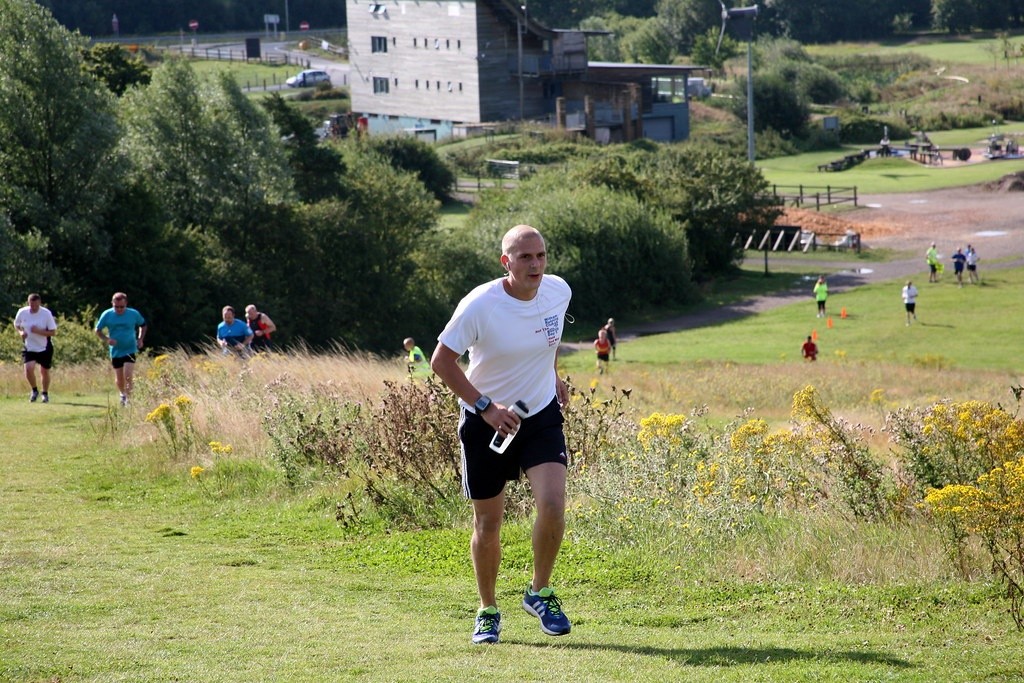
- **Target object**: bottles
[488,400,530,454]
[222,339,227,352]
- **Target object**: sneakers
[523,583,571,635]
[472,605,500,644]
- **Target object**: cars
[285,70,334,88]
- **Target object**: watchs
[474,394,492,416]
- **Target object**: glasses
[114,305,124,308]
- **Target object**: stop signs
[299,21,310,31]
[189,20,199,30]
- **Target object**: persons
[244,305,278,353]
[215,305,255,361]
[402,337,431,379]
[950,248,967,288]
[902,280,919,327]
[94,293,146,409]
[814,276,828,318]
[964,244,981,284]
[13,294,57,402]
[604,318,619,361]
[925,242,940,283]
[595,329,611,375]
[430,225,576,644]
[801,336,818,361]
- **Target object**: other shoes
[30,391,38,402]
[126,383,133,392]
[42,394,48,403]
[120,393,127,404]
